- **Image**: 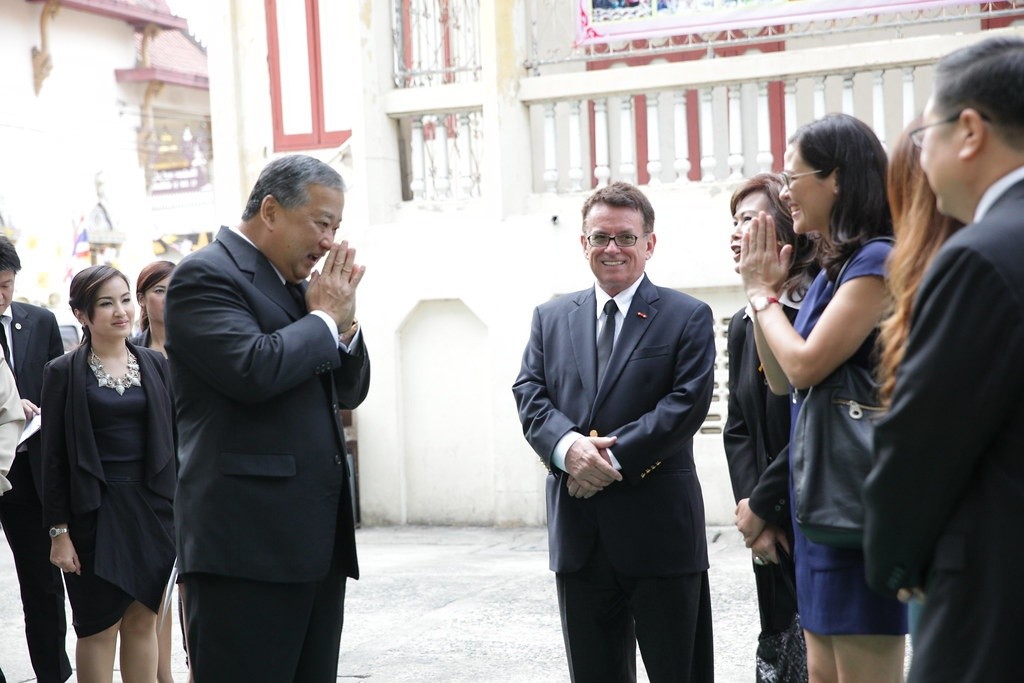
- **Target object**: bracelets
[338,319,358,342]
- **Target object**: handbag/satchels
[792,356,886,543]
[751,545,808,683]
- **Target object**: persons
[723,113,966,683]
[864,36,1024,683]
[164,155,371,683]
[511,182,717,683]
[0,236,177,683]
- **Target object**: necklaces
[90,348,141,396]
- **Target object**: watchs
[753,296,779,314]
[49,527,69,538]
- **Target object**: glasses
[584,232,650,248]
[908,111,991,149]
[782,170,823,190]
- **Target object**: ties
[597,299,619,391]
[0,322,12,371]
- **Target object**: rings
[754,556,764,565]
